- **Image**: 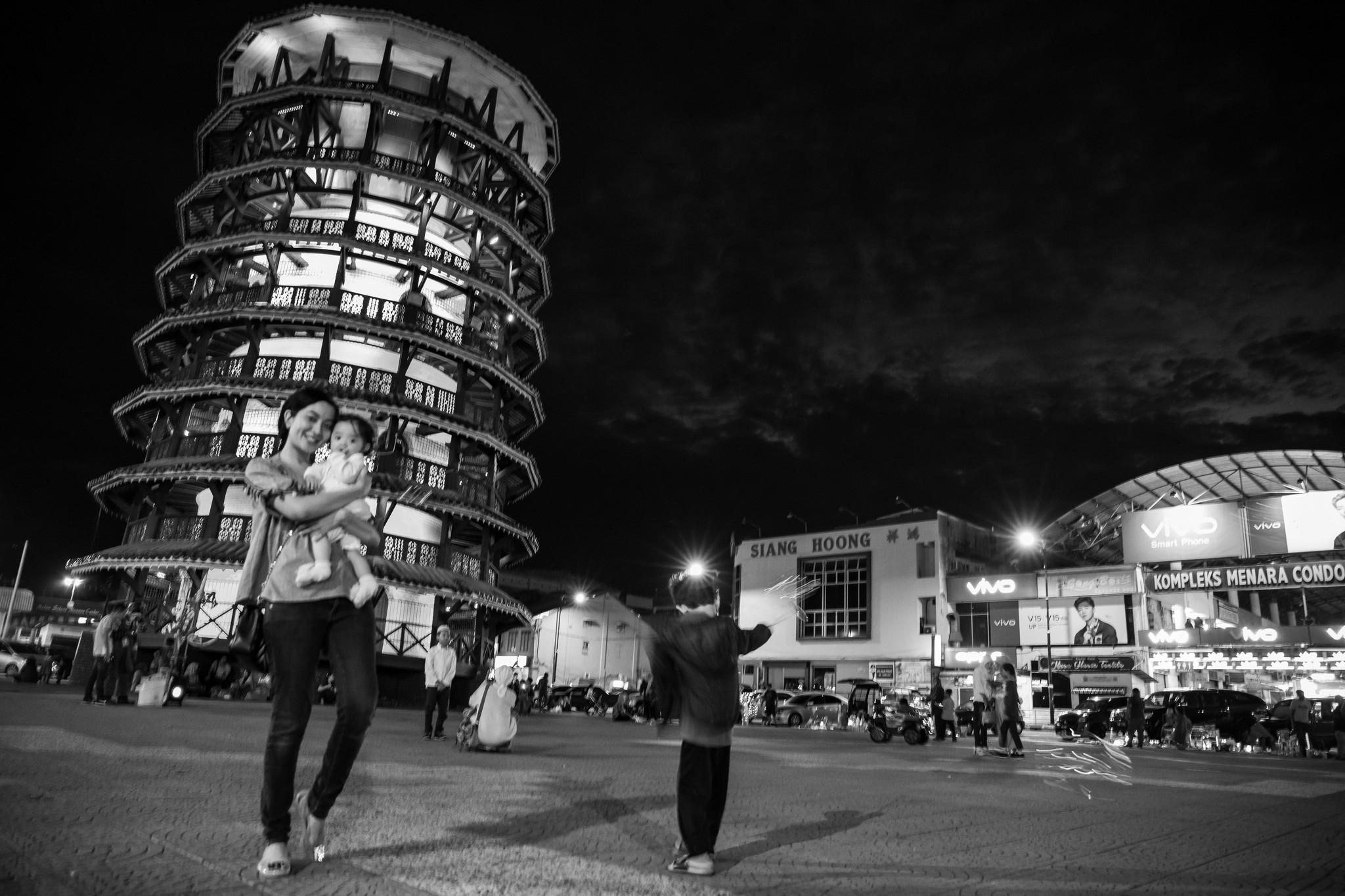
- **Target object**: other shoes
[257,848,292,879]
[296,790,326,863]
[992,748,1024,759]
[423,730,447,742]
[668,854,714,875]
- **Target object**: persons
[1289,690,1322,758]
[79,602,129,706]
[1145,706,1188,745]
[585,684,595,713]
[130,637,236,705]
[1124,687,1146,749]
[222,668,254,700]
[646,572,773,875]
[924,678,946,741]
[318,671,338,706]
[467,665,518,753]
[897,698,918,716]
[934,689,957,742]
[420,624,457,742]
[38,655,65,684]
[763,684,778,727]
[761,706,768,726]
[1190,712,1277,752]
[537,673,549,708]
[235,387,382,873]
[1074,597,1117,644]
[1324,696,1345,761]
[971,654,1025,760]
[293,412,386,613]
[515,677,534,714]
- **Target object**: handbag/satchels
[457,716,480,750]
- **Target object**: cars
[1055,688,1345,753]
[737,690,797,723]
[0,638,61,678]
[770,692,849,728]
[532,685,620,711]
[629,692,648,717]
[953,699,1026,735]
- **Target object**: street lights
[553,593,586,683]
[1019,530,1055,724]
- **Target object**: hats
[437,624,450,634]
[495,665,513,698]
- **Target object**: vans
[837,678,936,734]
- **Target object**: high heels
[973,745,989,756]
